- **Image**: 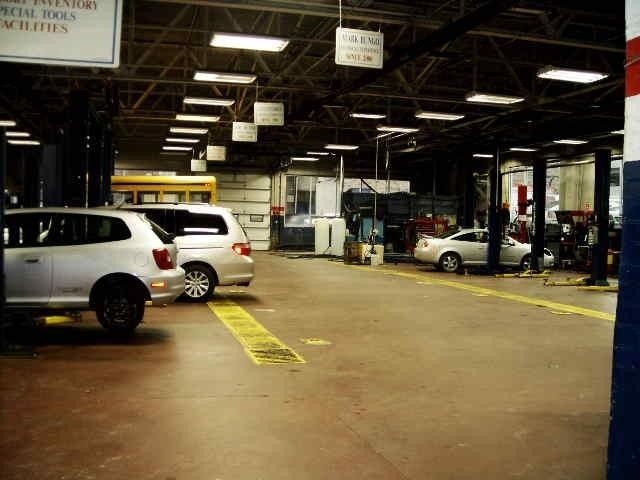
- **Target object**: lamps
[509,147,537,152]
[4,131,31,137]
[169,126,209,135]
[192,70,258,85]
[7,139,41,146]
[306,152,336,156]
[465,90,525,105]
[610,129,623,136]
[349,113,388,119]
[182,96,235,107]
[208,31,290,53]
[536,64,610,84]
[290,157,320,162]
[324,144,360,150]
[165,137,201,144]
[376,123,420,134]
[1,120,17,127]
[175,113,221,123]
[162,146,193,151]
[472,154,494,158]
[414,109,465,121]
[552,138,589,145]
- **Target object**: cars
[412,226,555,273]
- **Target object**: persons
[346,212,360,241]
[500,203,511,225]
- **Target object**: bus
[100,175,217,205]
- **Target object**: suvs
[4,203,254,334]
